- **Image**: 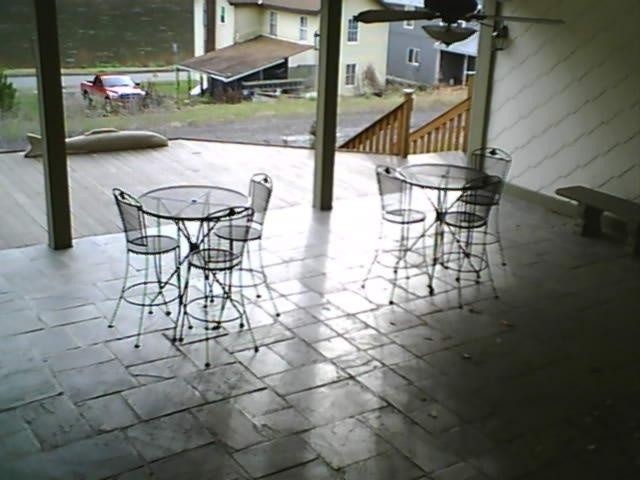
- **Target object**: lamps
[489,24,511,51]
[419,22,478,50]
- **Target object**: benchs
[555,185,640,244]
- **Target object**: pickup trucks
[79,73,149,114]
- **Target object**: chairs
[360,144,513,310]
[107,171,282,371]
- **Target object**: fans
[351,0,566,31]
